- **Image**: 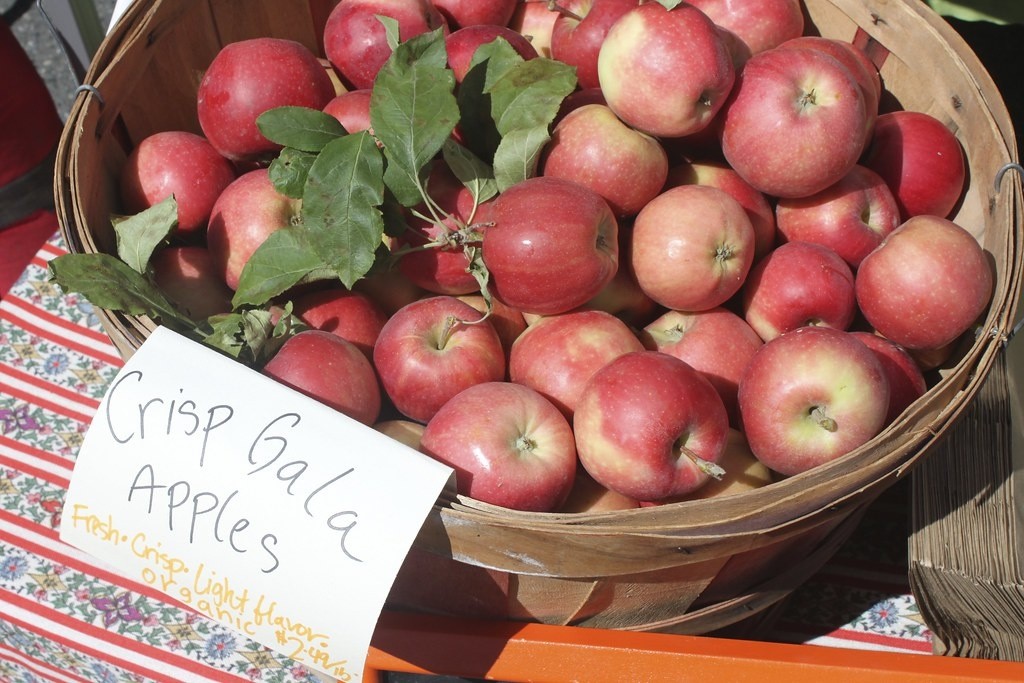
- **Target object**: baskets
[52,0,1024,637]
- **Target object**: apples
[119,0,995,516]
